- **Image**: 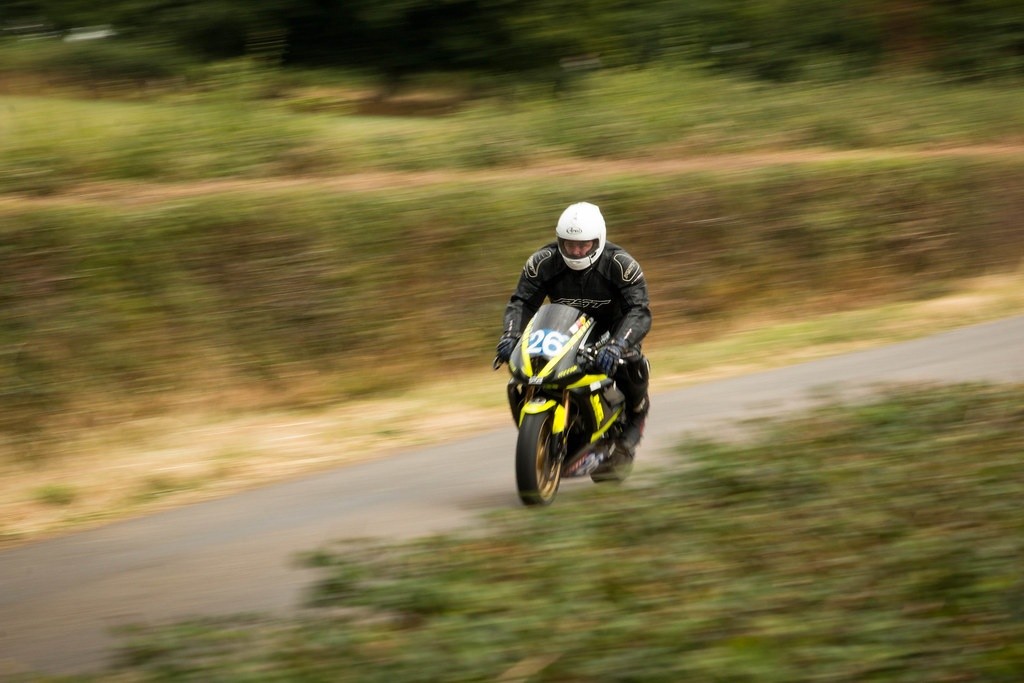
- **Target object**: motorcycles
[493,304,650,507]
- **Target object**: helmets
[555,202,607,270]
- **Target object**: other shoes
[616,398,649,451]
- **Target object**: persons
[495,201,651,453]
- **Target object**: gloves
[495,332,523,364]
[596,336,629,375]
